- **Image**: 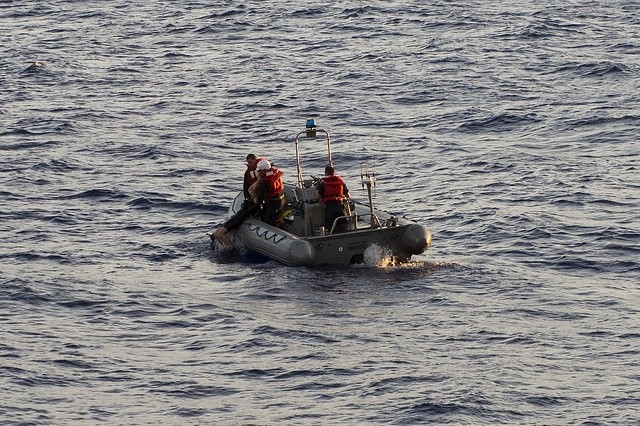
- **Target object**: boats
[227,118,433,269]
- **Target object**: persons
[243,154,266,197]
[210,197,259,246]
[317,165,350,231]
[255,160,285,214]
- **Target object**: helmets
[256,159,271,171]
